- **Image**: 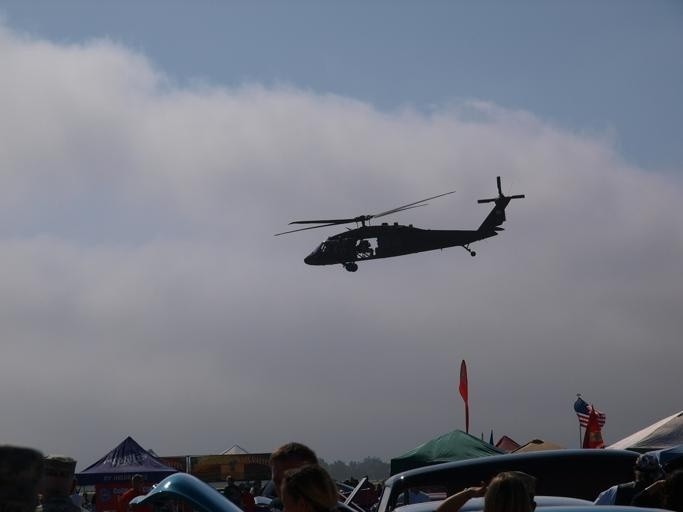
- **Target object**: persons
[357,238,374,257]
[224,478,263,512]
[595,456,683,511]
[437,471,535,512]
[121,475,153,512]
[0,446,86,512]
[272,442,339,508]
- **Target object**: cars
[129,446,682,512]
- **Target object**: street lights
[506,440,544,454]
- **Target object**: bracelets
[464,488,471,499]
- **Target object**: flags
[459,360,467,403]
[574,398,606,448]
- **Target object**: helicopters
[264,172,525,272]
